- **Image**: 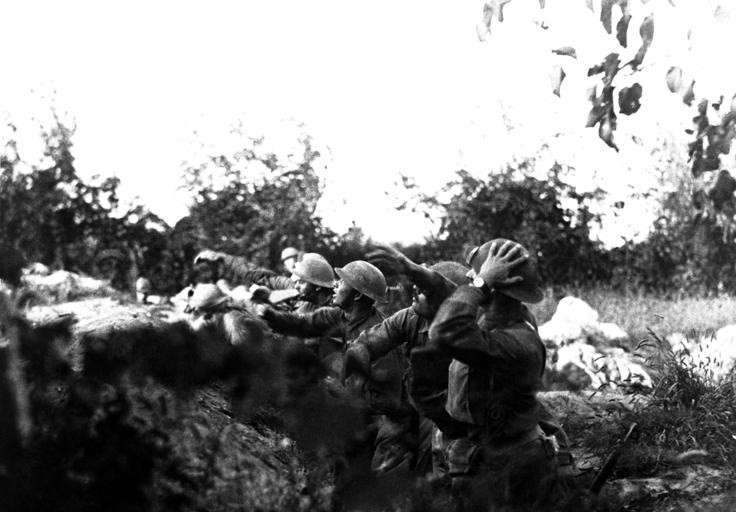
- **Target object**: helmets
[334,258,387,302]
[429,261,472,287]
[469,237,544,304]
[287,255,337,291]
[280,247,297,261]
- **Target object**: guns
[581,421,638,512]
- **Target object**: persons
[365,238,586,512]
[328,262,484,512]
[188,247,420,512]
